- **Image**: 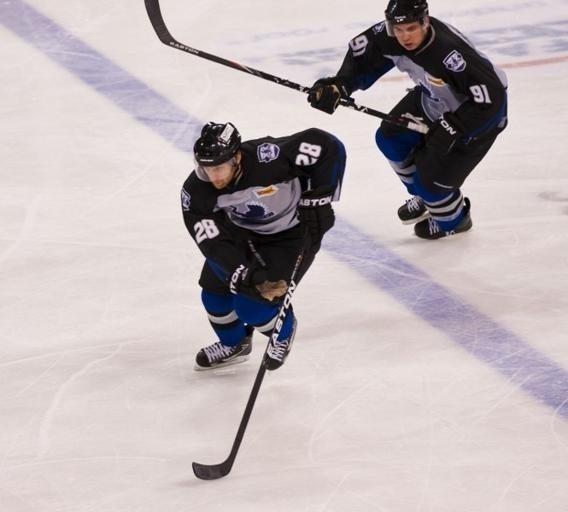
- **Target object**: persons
[307,0,508,239]
[182,122,347,370]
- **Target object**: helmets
[385,0,429,24]
[194,122,242,166]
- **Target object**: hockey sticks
[192,237,305,479]
[144,0,429,134]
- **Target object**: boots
[398,197,427,220]
[415,207,473,240]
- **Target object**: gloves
[240,266,287,303]
[298,192,335,239]
[425,107,475,155]
[308,77,352,114]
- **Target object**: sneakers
[196,334,254,367]
[262,317,297,370]
